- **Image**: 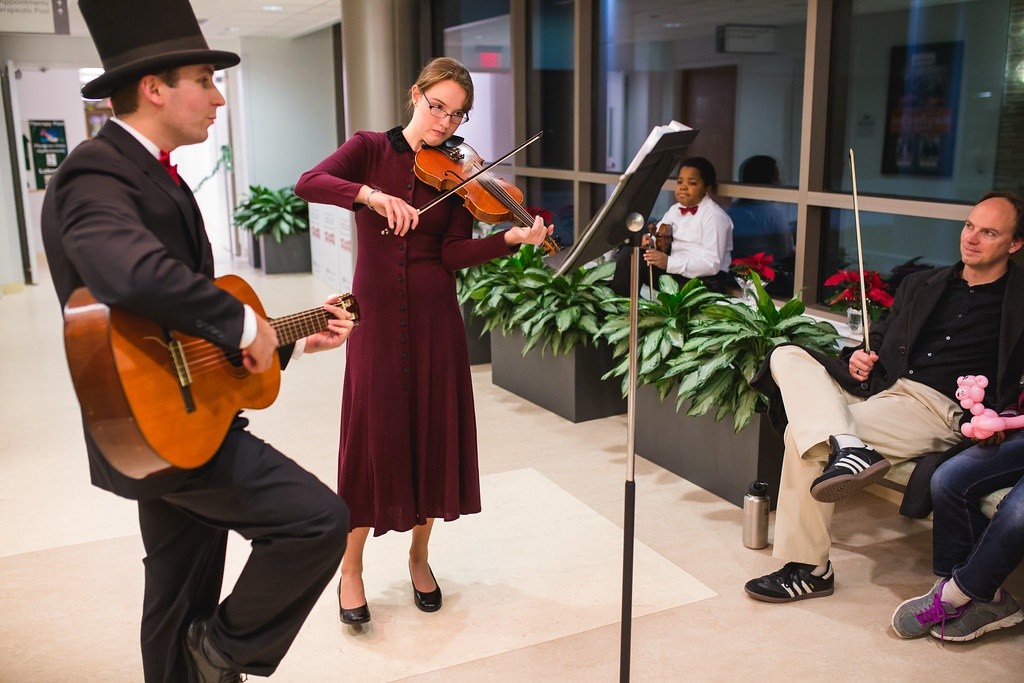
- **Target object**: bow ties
[677,206,699,215]
[159,149,180,187]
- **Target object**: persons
[724,154,799,278]
[739,189,1024,604]
[295,55,555,633]
[34,0,350,683]
[641,155,736,295]
[891,399,1024,646]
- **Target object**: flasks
[742,480,771,550]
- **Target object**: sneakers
[891,577,964,639]
[930,588,1024,642]
[744,559,834,603]
[809,435,891,503]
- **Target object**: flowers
[822,265,892,310]
[729,252,776,280]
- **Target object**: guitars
[62,270,362,480]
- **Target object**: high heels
[337,574,372,625]
[408,560,443,612]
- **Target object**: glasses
[420,88,469,125]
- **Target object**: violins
[411,132,564,260]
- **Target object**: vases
[846,307,870,336]
[740,278,766,307]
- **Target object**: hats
[78,0,241,99]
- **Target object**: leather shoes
[183,609,246,683]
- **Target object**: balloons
[955,374,1024,440]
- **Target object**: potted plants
[455,242,839,515]
[231,180,312,274]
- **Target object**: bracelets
[365,188,383,212]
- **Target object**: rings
[855,369,861,375]
